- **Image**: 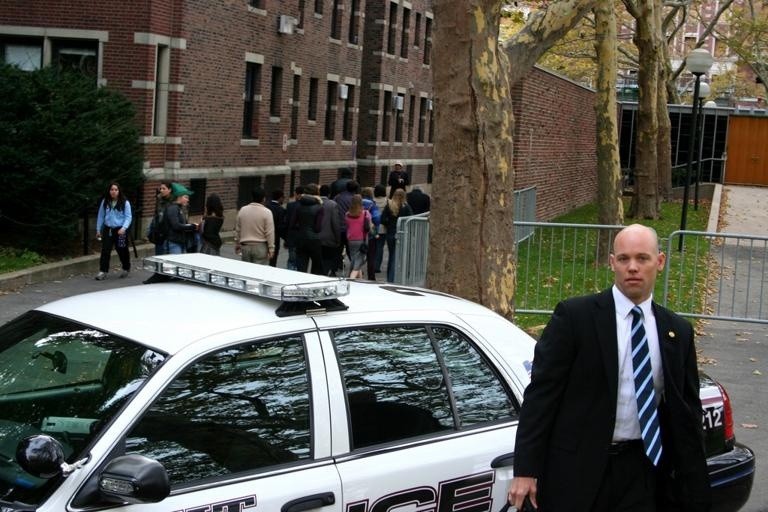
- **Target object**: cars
[0,251,754,512]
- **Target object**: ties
[631,305,664,467]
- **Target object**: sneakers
[96,271,106,280]
[120,269,130,277]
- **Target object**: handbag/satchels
[364,217,370,232]
[148,210,168,245]
[359,243,368,254]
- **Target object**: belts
[608,440,643,458]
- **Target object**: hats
[170,182,195,201]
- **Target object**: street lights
[676,48,713,253]
[703,100,717,108]
[694,82,711,210]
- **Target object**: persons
[95,183,132,279]
[144,162,431,284]
[507,223,714,512]
